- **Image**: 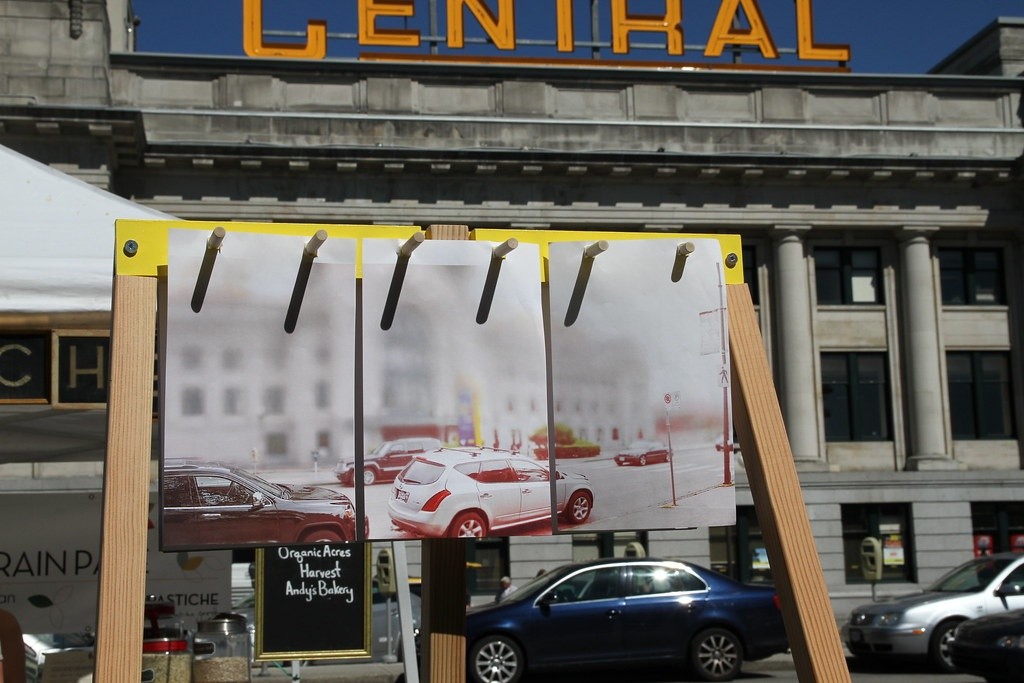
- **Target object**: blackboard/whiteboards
[254,541,372,662]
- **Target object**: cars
[467,557,789,683]
[840,552,1024,672]
[613,441,673,466]
[714,434,733,452]
[947,606,1024,683]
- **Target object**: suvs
[164,455,369,542]
[333,437,446,487]
[387,446,596,537]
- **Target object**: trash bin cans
[141,637,193,682]
[193,616,252,683]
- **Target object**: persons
[643,574,679,593]
[496,577,518,599]
[537,569,551,603]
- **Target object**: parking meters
[860,536,882,581]
[623,542,646,558]
